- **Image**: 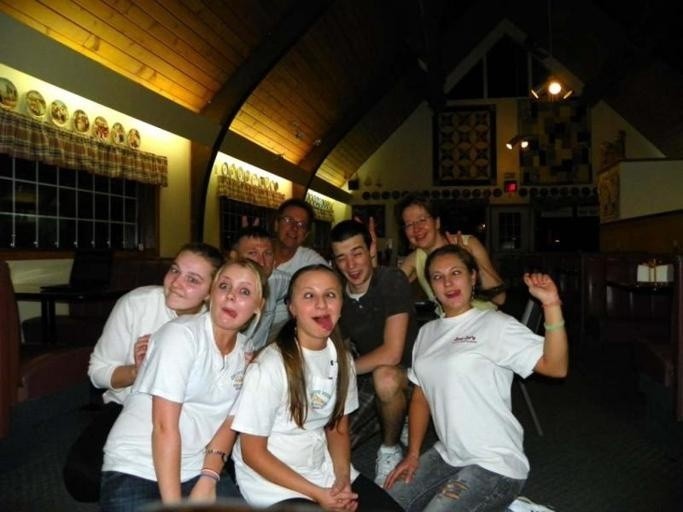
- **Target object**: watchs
[204,447,228,464]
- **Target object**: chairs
[498,268,550,437]
[0,257,101,438]
[577,256,681,462]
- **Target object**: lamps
[528,1,575,104]
[505,134,531,151]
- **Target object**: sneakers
[374,445,403,487]
[509,495,556,512]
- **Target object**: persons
[228,226,294,361]
[242,197,330,348]
[100,259,270,511]
[354,195,507,320]
[228,264,405,510]
[382,245,570,511]
[327,220,423,488]
[86,242,223,443]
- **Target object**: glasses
[279,215,310,232]
[404,215,436,229]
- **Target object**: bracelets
[539,299,562,307]
[201,468,222,483]
[541,320,566,329]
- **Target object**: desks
[5,259,174,345]
